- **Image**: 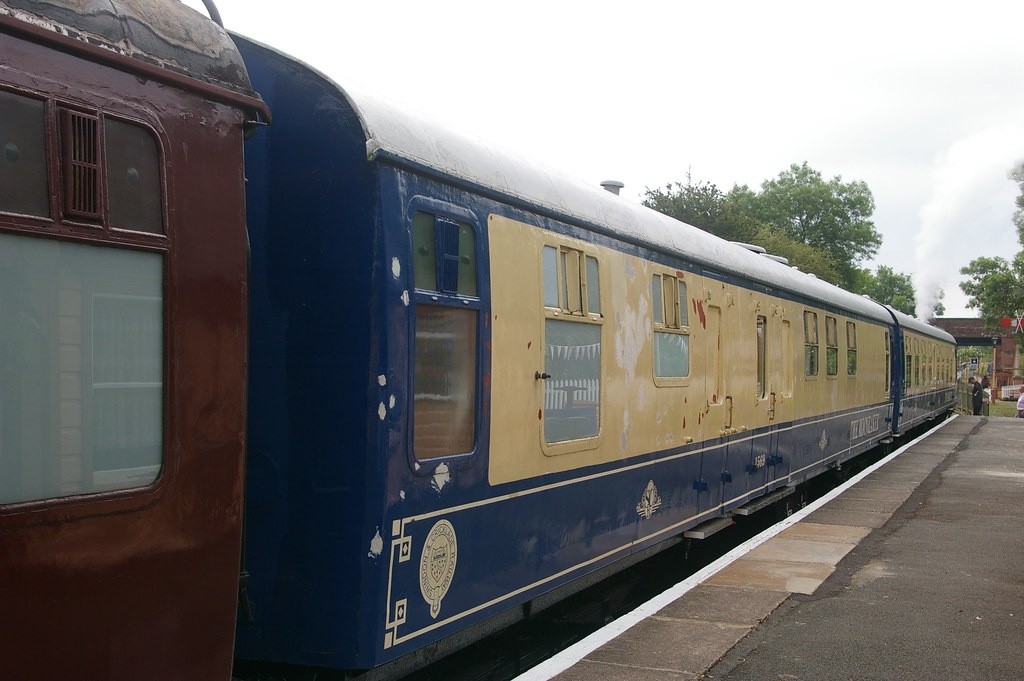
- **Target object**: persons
[1017,386,1024,418]
[968,377,983,416]
[981,376,990,389]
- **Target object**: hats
[968,376,972,382]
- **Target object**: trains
[0,1,958,681]
